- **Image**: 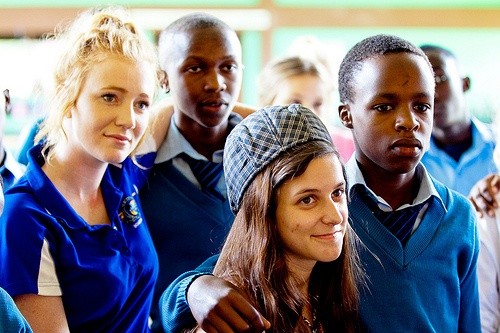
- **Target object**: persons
[140,14,246,300]
[187,46,500,333]
[0,87,33,333]
[0,7,257,333]
[158,36,481,333]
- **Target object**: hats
[222,103,334,215]
